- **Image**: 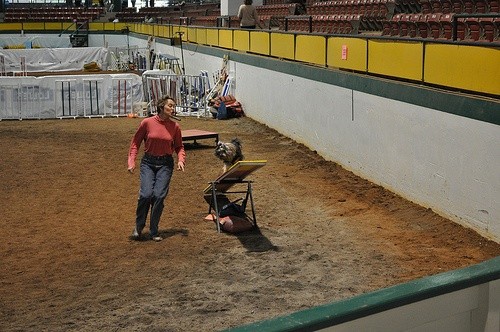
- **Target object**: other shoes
[150,234,163,242]
[131,228,141,240]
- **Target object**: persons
[128,97,185,241]
[237,0,265,29]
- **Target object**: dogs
[213,136,246,174]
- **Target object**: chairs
[109,4,220,26]
[225,0,500,42]
[4,6,104,22]
